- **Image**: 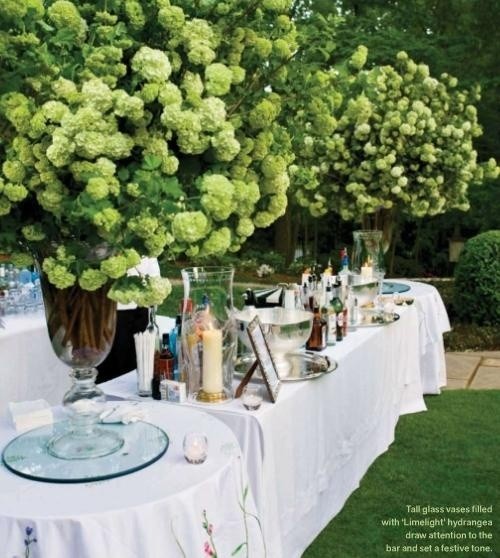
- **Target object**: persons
[74,239,163,387]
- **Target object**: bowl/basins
[234,308,314,350]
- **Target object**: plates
[235,353,339,382]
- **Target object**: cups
[159,380,189,403]
[381,291,415,323]
[183,432,209,465]
[243,384,262,411]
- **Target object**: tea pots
[351,230,389,279]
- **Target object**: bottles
[0,263,44,314]
[158,333,174,382]
[296,254,351,351]
[243,288,256,311]
[181,265,237,405]
[144,295,216,381]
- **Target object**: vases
[32,243,127,462]
